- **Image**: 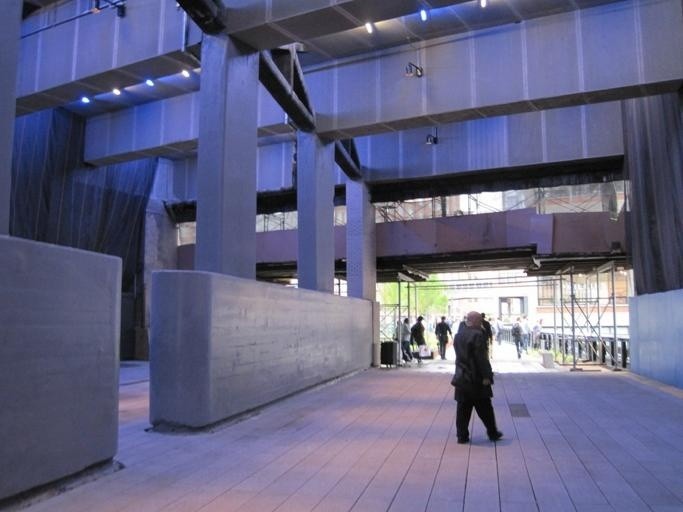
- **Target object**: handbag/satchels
[419,345,431,356]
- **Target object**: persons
[448,310,505,444]
[392,311,544,363]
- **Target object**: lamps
[426,134,438,146]
[91,0,124,17]
[406,61,423,78]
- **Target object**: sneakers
[490,432,502,441]
[458,437,469,443]
